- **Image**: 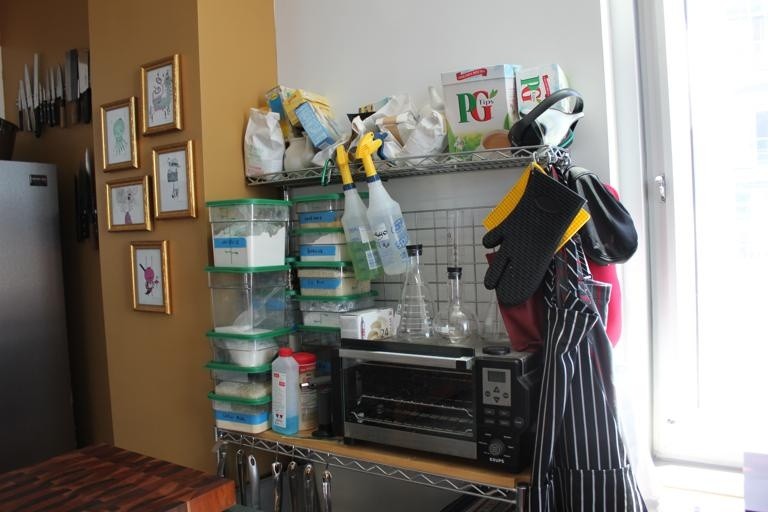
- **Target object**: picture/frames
[152,139,197,219]
[139,53,183,135]
[128,239,171,316]
[99,95,140,172]
[105,175,154,232]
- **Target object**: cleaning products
[355,132,414,276]
[336,144,385,282]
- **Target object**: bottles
[393,244,434,342]
[292,352,318,437]
[368,176,411,277]
[341,183,384,281]
[432,266,477,344]
[479,286,506,343]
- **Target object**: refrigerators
[1,160,80,476]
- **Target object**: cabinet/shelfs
[213,145,571,512]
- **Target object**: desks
[1,442,236,511]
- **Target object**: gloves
[479,167,588,308]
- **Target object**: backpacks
[563,162,638,267]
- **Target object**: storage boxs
[208,393,272,434]
[297,323,341,349]
[292,262,372,295]
[205,363,272,398]
[292,192,370,228]
[209,335,278,367]
[514,61,572,121]
[204,197,292,268]
[291,289,378,327]
[294,227,361,262]
[205,264,289,334]
[440,64,519,161]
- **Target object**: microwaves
[340,338,540,474]
